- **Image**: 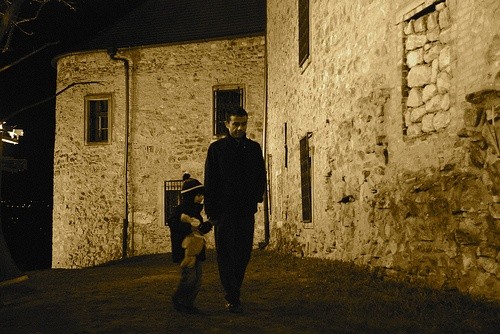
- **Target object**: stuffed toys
[181,213,204,268]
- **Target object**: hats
[181,174,205,206]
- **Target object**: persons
[203,107,267,311]
[170,173,213,314]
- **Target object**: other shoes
[173,299,201,313]
[225,300,241,313]
